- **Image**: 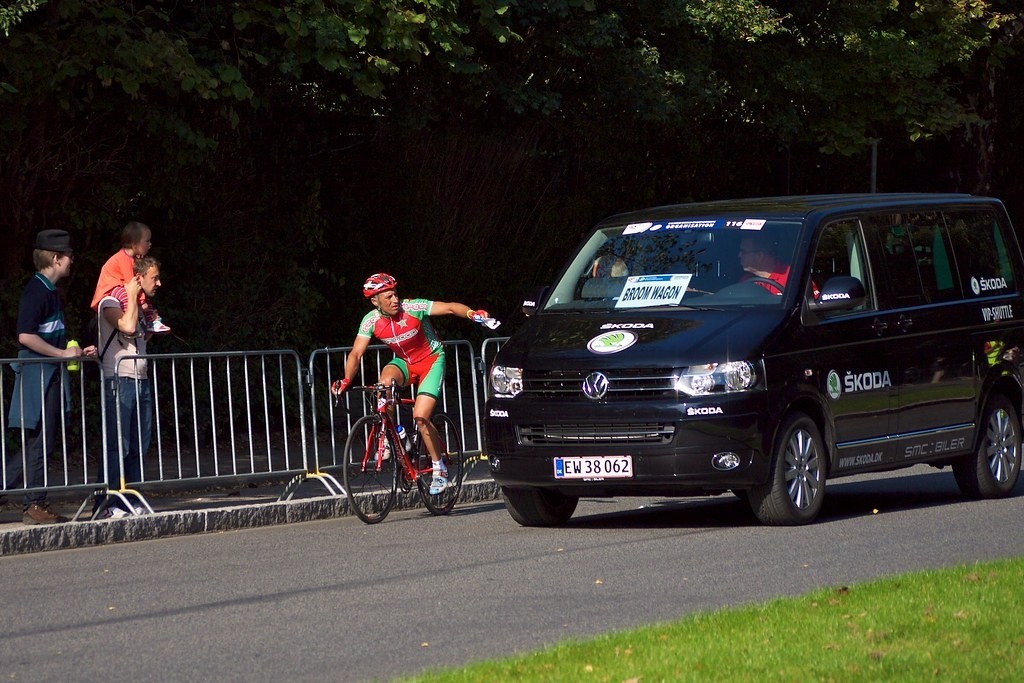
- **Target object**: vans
[480,192,1024,525]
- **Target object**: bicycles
[332,378,465,526]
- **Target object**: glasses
[736,247,758,259]
[59,254,75,262]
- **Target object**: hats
[35,229,74,252]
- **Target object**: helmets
[363,273,397,298]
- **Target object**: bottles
[395,425,411,451]
[412,423,419,448]
[67,340,79,370]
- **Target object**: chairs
[816,238,847,281]
[688,241,728,277]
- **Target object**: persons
[0,230,98,525]
[738,231,820,300]
[91,222,171,519]
[331,273,490,493]
[593,256,629,277]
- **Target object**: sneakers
[374,429,411,460]
[95,507,127,519]
[123,330,139,339]
[22,502,68,524]
[430,468,448,494]
[146,321,170,332]
[131,506,149,516]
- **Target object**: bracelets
[467,310,474,319]
